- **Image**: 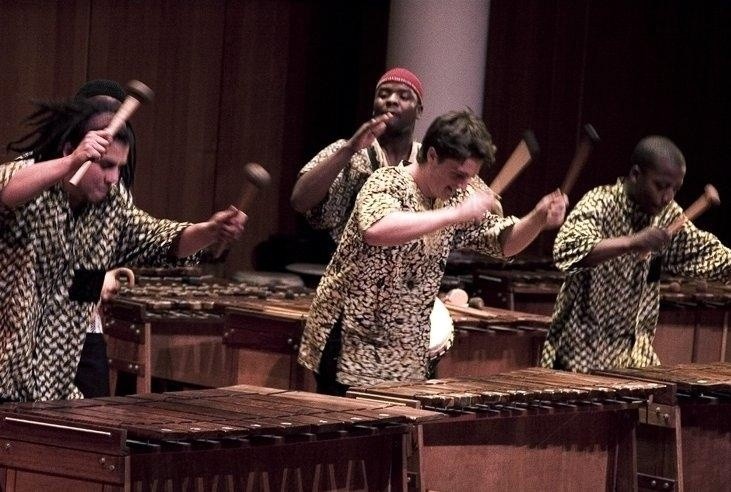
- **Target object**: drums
[285,263,326,286]
[426,296,456,375]
[232,268,304,293]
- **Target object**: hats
[375,67,424,106]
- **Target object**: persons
[536,131,730,379]
[67,77,137,406]
[292,102,570,399]
[285,62,439,383]
[0,93,248,406]
